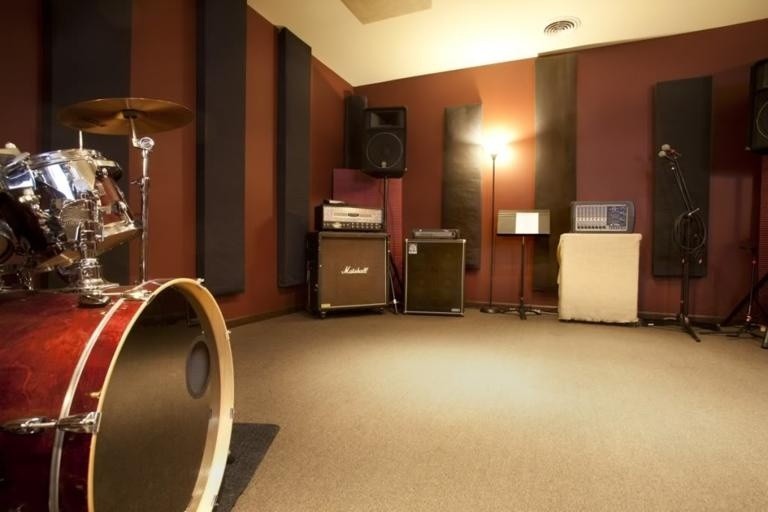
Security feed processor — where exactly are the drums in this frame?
[1,278,234,510]
[3,149,141,274]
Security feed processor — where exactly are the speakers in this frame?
[403,239,467,316]
[305,231,386,318]
[747,58,768,156]
[360,106,407,179]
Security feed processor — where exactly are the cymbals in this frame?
[61,98,194,137]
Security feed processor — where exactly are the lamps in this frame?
[480,142,511,316]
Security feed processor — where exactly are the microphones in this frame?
[657,150,674,162]
[661,143,681,157]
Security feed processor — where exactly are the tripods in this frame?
[384,177,402,314]
[500,236,541,319]
[643,163,720,341]
[700,247,765,339]
